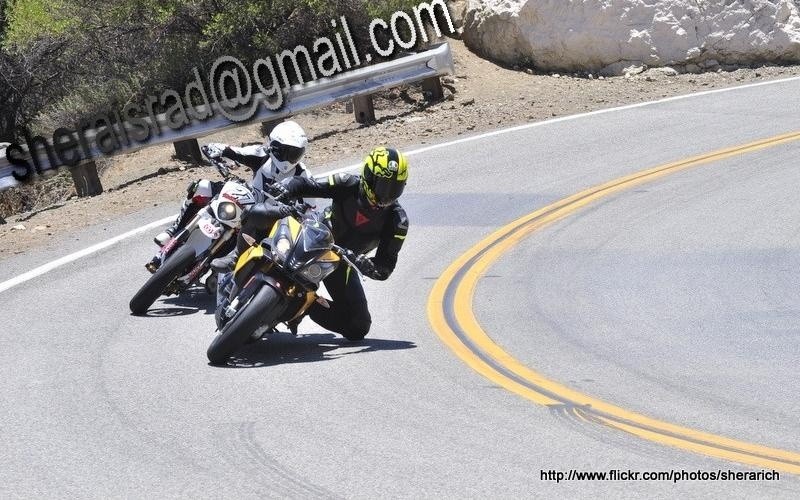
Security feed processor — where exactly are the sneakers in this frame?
[154,224,178,248]
[211,250,240,268]
[288,309,305,335]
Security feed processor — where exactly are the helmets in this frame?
[361,146,409,208]
[268,120,307,174]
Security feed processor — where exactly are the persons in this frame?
[209,146,410,340]
[153,121,316,295]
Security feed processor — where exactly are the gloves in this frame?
[354,253,376,275]
[270,182,292,203]
[205,142,229,158]
